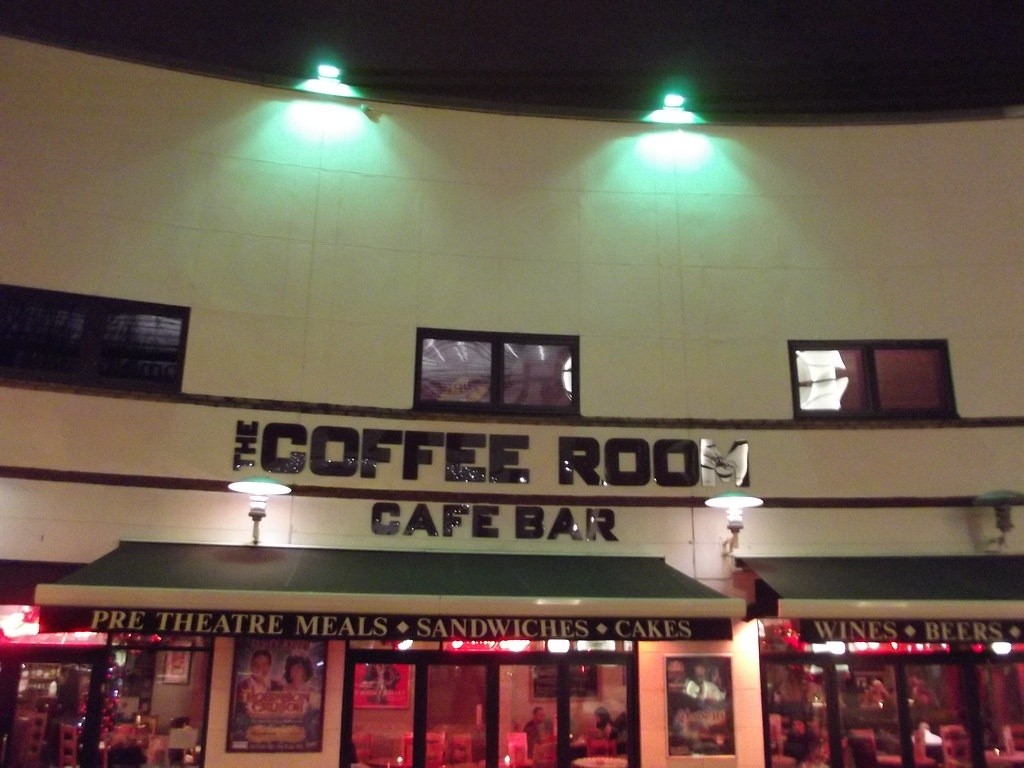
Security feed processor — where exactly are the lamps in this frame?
[227,477,292,543]
[976,489,1024,544]
[705,492,764,557]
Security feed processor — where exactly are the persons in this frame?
[39,665,204,768]
[668,658,732,756]
[767,673,993,768]
[521,706,628,759]
[233,650,321,742]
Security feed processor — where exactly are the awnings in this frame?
[33,541,746,642]
[736,556,1024,621]
